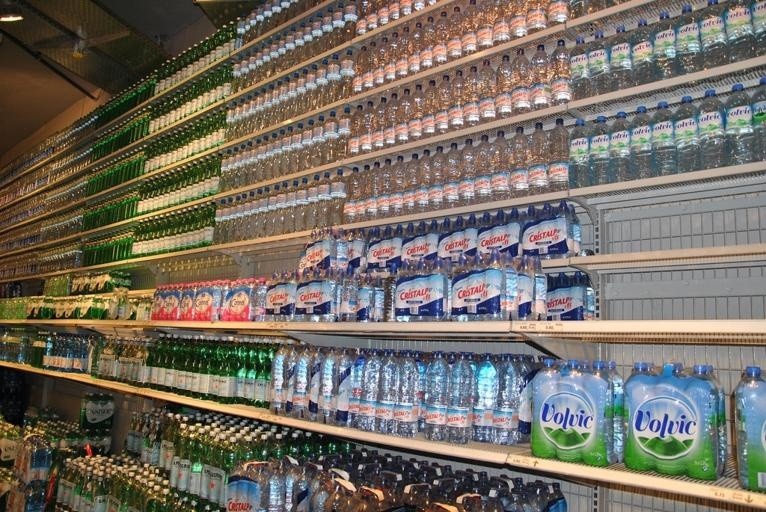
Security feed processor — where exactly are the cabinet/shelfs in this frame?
[0,1,766,512]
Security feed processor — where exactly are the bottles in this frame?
[0,0,765,512]
